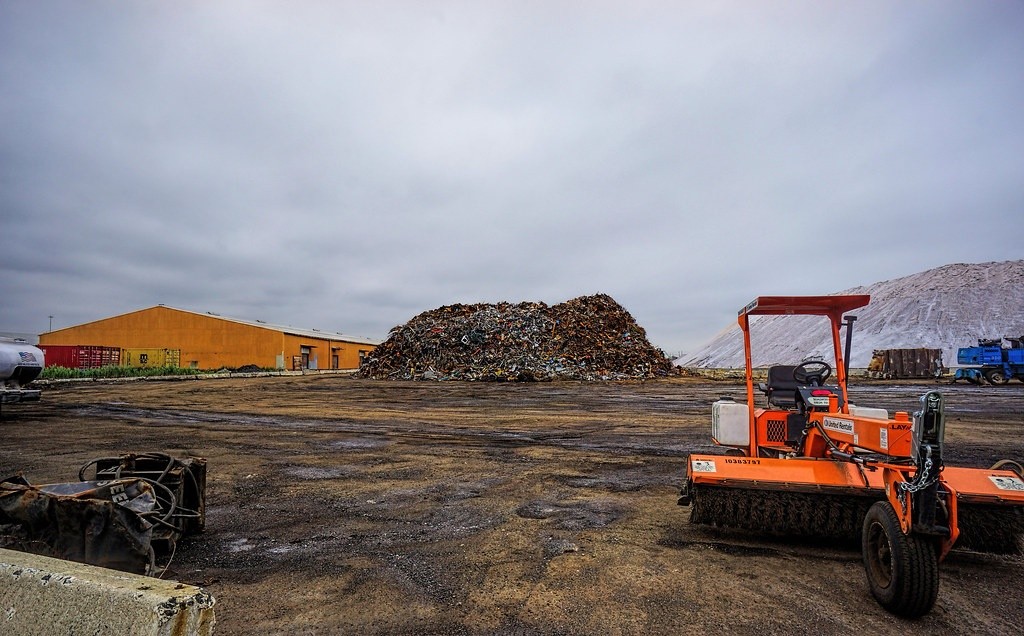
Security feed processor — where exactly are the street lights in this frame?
[49,315,54,333]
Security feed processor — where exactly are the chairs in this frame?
[764,364,810,408]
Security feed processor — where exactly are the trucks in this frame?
[956,335,1024,386]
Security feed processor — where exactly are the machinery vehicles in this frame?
[680,294,1024,622]
